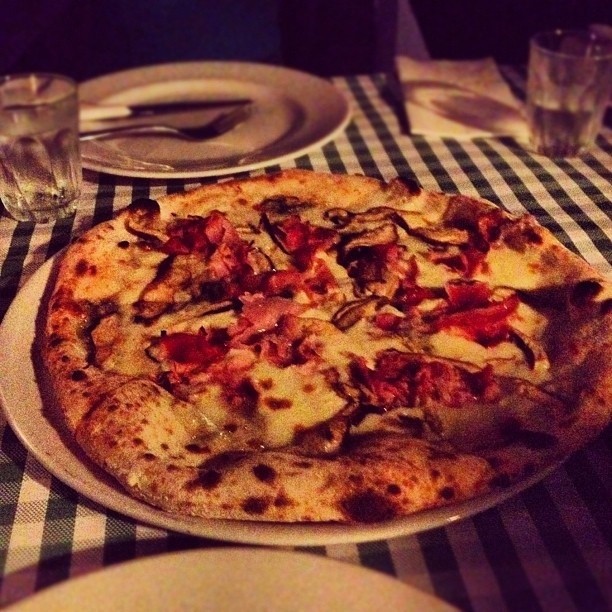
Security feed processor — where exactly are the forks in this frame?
[78,106,254,142]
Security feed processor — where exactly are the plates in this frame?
[78,61,355,179]
[1,546,466,611]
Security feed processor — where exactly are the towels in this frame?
[391,53,529,139]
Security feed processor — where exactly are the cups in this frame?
[0,71,80,221]
[526,23,612,156]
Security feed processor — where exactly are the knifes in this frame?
[78,95,255,121]
